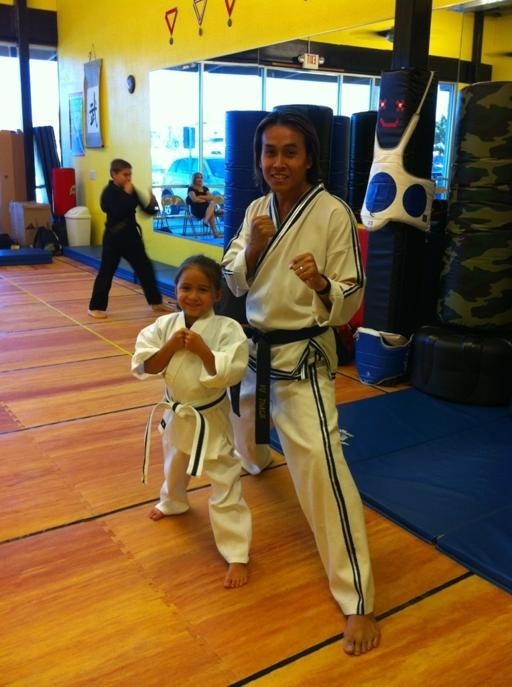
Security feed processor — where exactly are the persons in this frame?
[188,171,221,237]
[129,253,253,588]
[86,157,176,319]
[220,109,384,657]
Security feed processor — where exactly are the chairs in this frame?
[153,195,225,237]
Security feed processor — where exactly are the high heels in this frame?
[203,222,208,227]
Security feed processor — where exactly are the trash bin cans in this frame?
[64,206,91,246]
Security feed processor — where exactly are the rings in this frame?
[299,265,303,272]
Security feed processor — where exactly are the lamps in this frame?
[297,54,325,69]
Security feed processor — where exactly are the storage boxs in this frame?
[8,201,52,249]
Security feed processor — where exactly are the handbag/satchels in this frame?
[186,192,198,205]
[33,223,67,256]
[0,234,18,249]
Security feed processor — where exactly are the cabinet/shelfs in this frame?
[0,133,27,235]
